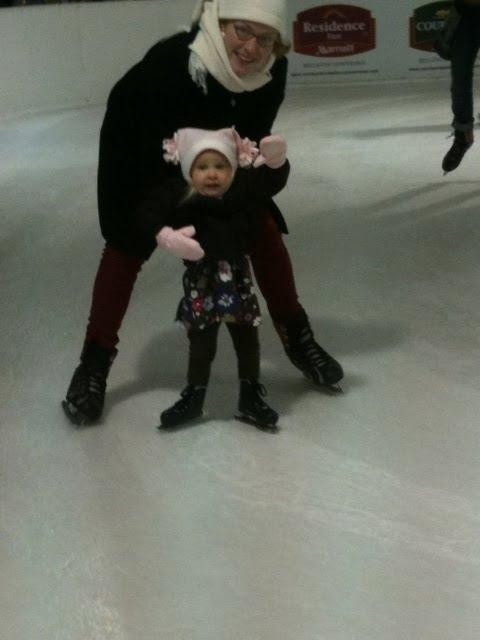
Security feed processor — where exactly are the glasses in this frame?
[221,23,281,50]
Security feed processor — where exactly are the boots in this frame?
[64,340,120,422]
[442,120,475,171]
[270,307,344,387]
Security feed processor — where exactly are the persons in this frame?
[62,0,343,426]
[438,0,480,175]
[156,121,292,434]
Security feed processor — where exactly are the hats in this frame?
[171,126,239,186]
[214,0,292,55]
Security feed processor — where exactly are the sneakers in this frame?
[237,381,279,425]
[159,387,207,429]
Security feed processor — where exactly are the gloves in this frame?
[156,223,206,263]
[259,132,288,170]
